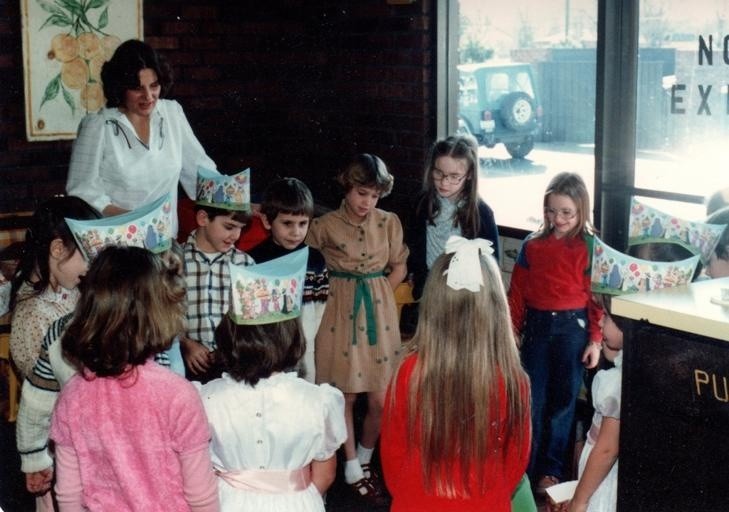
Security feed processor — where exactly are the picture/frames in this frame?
[20,0,145,142]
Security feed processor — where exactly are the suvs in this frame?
[454,60,545,158]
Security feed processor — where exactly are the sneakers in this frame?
[535,477,558,493]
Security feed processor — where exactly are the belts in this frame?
[529,310,588,318]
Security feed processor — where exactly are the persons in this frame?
[565,188,728,512]
[9,192,105,384]
[190,246,349,512]
[380,235,533,511]
[177,164,257,381]
[16,189,179,511]
[404,136,501,338]
[509,170,604,497]
[303,152,411,505]
[48,244,219,512]
[65,39,222,218]
[244,177,330,386]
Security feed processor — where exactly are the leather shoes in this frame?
[345,463,385,501]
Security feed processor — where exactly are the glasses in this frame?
[432,169,468,185]
[544,207,579,219]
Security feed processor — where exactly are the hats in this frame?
[196,165,251,212]
[628,199,728,265]
[591,234,701,296]
[65,192,171,265]
[228,247,309,325]
